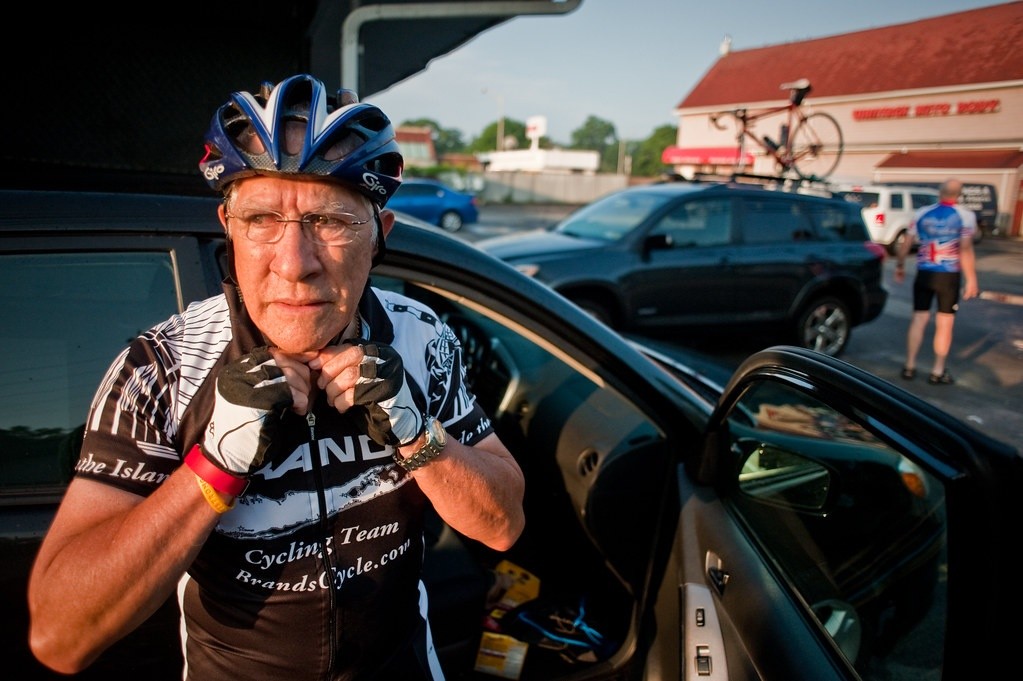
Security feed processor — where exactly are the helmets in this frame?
[197,71,404,211]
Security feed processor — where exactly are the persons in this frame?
[26,73,526,681]
[893,179,978,385]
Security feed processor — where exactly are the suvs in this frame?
[831,183,980,254]
[0,186,1023,680]
[467,179,891,357]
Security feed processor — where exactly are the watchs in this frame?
[896,261,903,269]
[392,415,447,471]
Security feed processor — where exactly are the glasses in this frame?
[223,210,377,246]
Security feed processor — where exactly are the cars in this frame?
[389,179,479,232]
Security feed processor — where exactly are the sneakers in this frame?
[928,367,956,385]
[900,365,918,380]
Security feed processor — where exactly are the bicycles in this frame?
[701,78,843,181]
